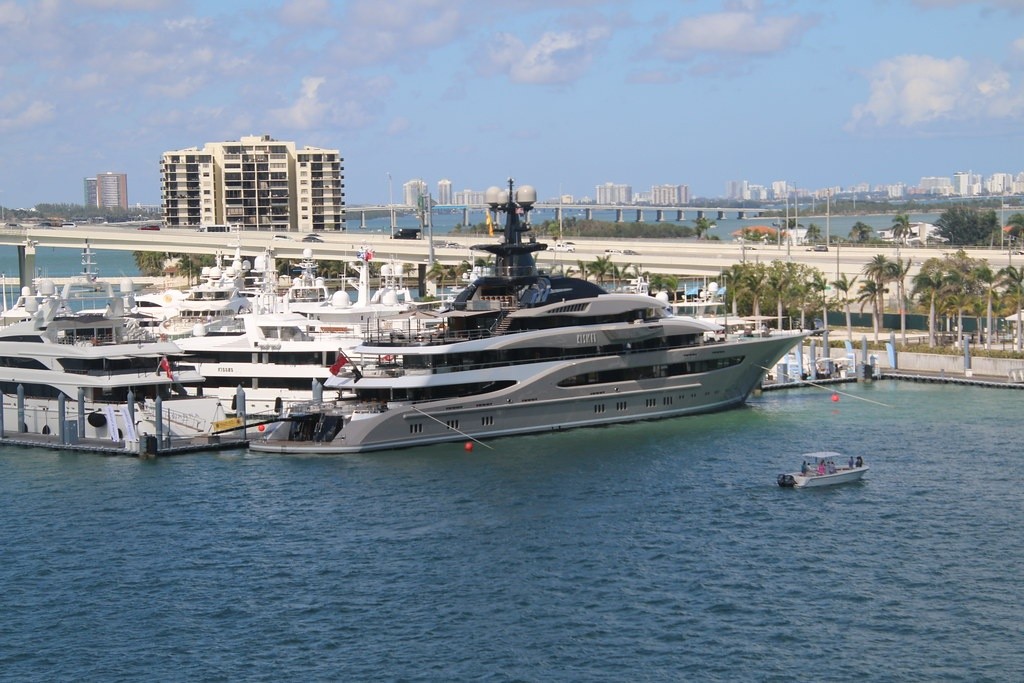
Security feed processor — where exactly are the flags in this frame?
[330,354,348,375]
[160,357,174,381]
[357,251,366,259]
[365,252,373,262]
[486,208,495,236]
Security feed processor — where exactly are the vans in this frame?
[197,225,231,232]
[390,228,421,240]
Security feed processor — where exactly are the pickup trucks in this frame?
[431,238,466,248]
[548,243,575,252]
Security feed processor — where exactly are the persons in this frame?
[801,456,864,475]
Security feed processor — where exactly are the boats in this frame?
[1,177,835,459]
[777,451,870,488]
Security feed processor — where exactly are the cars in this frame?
[306,233,320,237]
[137,225,160,230]
[302,237,323,243]
[273,234,294,241]
[740,246,757,251]
[805,244,828,252]
[605,249,622,254]
[624,250,640,254]
